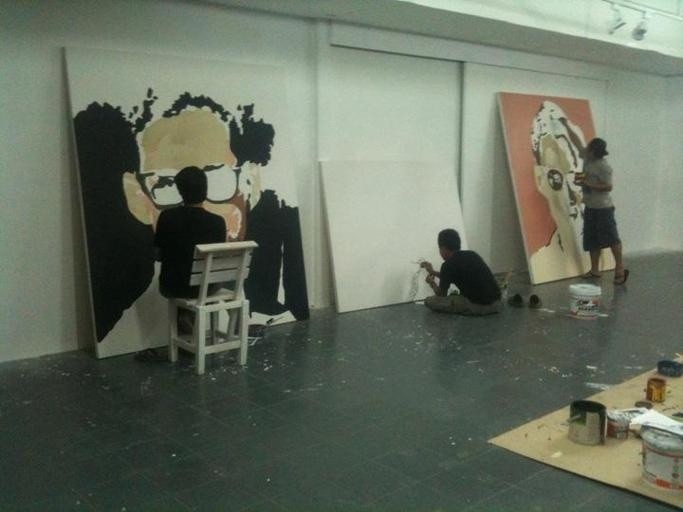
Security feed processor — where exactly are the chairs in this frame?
[168,241,259,376]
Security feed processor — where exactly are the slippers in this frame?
[529,295,542,308]
[508,294,524,307]
[614,269,629,284]
[584,270,602,278]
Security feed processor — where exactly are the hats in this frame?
[588,138,609,155]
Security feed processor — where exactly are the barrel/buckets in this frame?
[568,400,606,445]
[647,378,666,404]
[640,417,683,492]
[607,412,630,439]
[569,284,602,317]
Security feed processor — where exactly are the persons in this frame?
[558,116,630,286]
[420,228,505,314]
[97,93,298,354]
[154,166,227,353]
[529,100,616,282]
